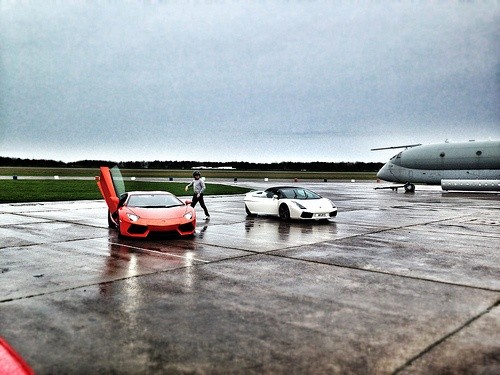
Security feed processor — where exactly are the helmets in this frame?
[193,171,202,177]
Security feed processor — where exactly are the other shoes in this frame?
[205,215,210,220]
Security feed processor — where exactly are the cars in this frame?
[94,165,197,241]
[244,186,338,223]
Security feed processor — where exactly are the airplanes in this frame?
[369,141,500,197]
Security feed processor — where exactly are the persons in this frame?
[184,171,211,220]
